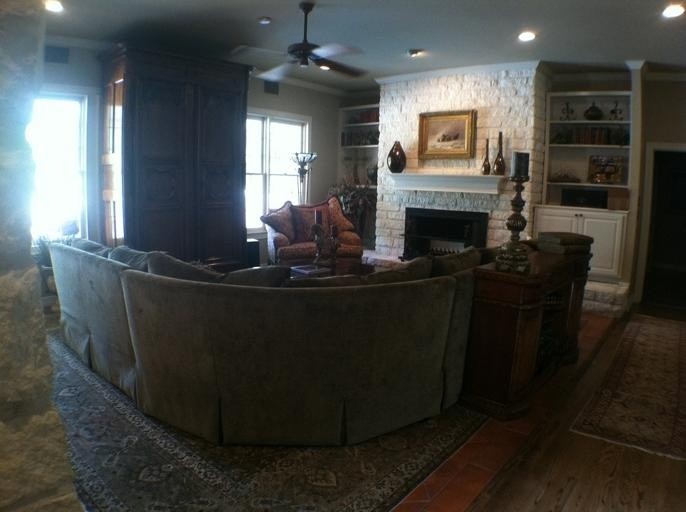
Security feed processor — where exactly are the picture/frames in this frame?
[417,109,477,160]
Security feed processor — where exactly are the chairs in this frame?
[259,196,349,265]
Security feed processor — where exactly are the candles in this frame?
[510,151,531,178]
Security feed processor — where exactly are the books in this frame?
[548,125,628,144]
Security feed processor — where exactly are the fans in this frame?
[229,0,368,86]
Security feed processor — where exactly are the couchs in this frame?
[44,235,540,446]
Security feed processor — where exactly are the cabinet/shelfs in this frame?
[461,251,593,419]
[99,42,255,273]
[541,88,630,213]
[333,105,380,251]
[531,203,628,281]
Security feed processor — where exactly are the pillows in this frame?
[260,195,357,241]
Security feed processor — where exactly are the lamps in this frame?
[289,152,317,203]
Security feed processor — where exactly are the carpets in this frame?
[43,329,493,512]
[570,310,686,463]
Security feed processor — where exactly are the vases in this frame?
[480,139,490,174]
[492,130,507,175]
[388,141,408,174]
